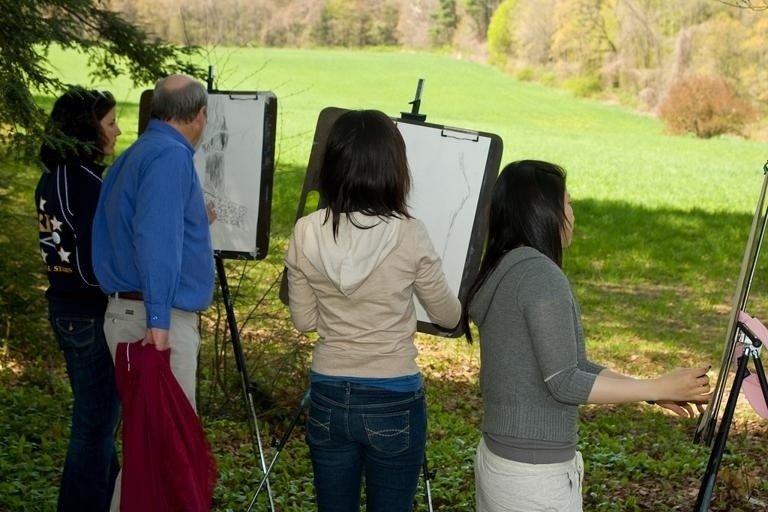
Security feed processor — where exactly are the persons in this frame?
[286,110,463,512]
[34,90,215,512]
[92,76,216,512]
[463,160,711,512]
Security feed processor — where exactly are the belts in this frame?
[111,291,144,300]
